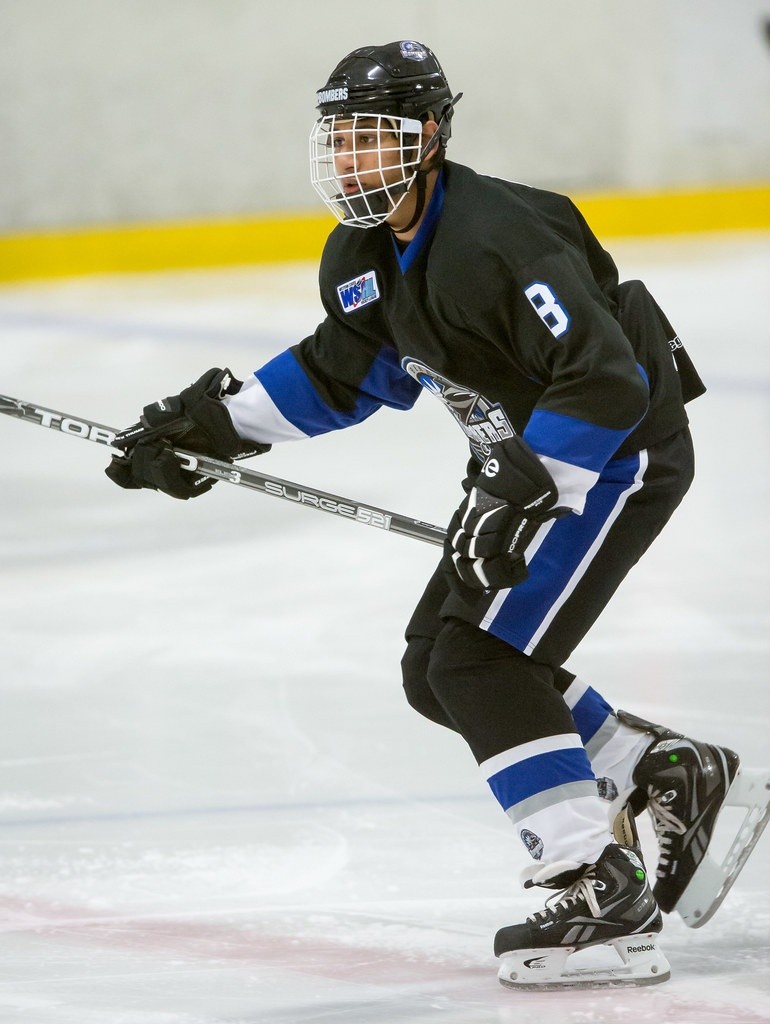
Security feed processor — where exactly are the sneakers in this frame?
[492,802,672,992]
[612,708,770,928]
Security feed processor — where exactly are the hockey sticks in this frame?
[0,394,448,549]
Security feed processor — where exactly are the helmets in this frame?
[316,41,455,143]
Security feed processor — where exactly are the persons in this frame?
[104,41,770,990]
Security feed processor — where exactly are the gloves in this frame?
[104,365,273,501]
[441,433,559,605]
